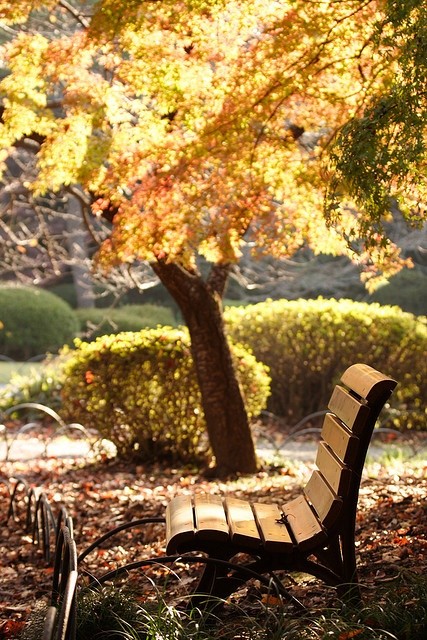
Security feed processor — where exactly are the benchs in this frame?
[166,363,397,610]
